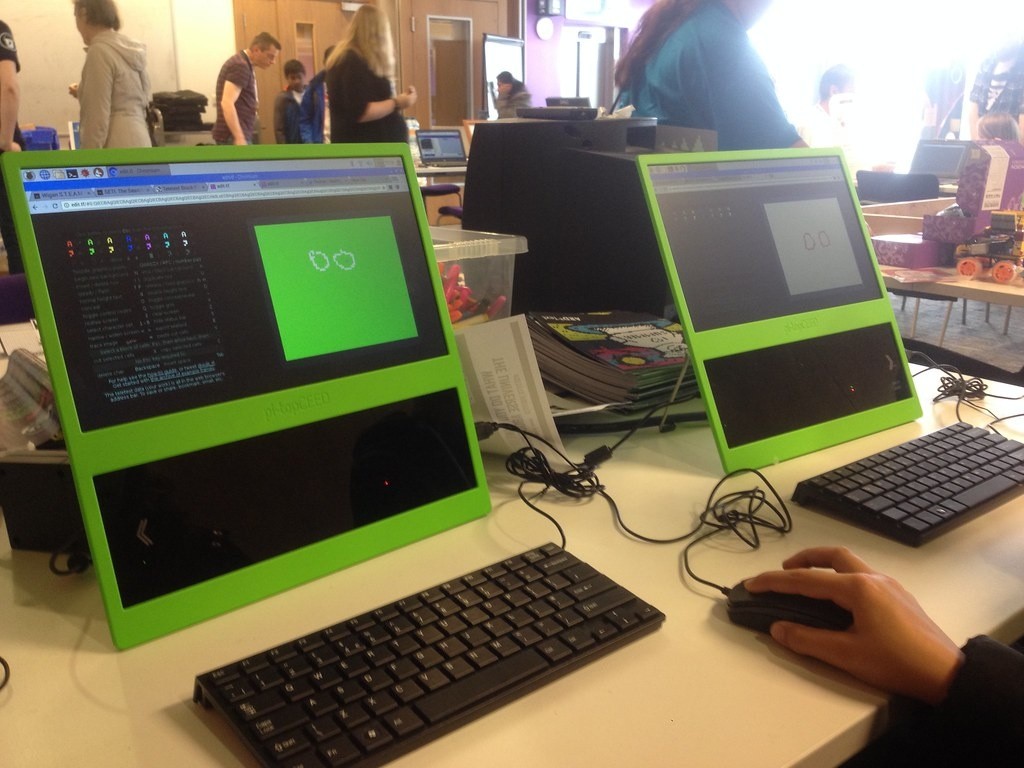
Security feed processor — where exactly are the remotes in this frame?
[516,106,598,120]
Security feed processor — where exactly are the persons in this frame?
[69,0,152,149]
[273,59,314,144]
[743,545,1024,768]
[615,0,810,152]
[495,71,531,119]
[921,42,1024,216]
[212,32,281,145]
[299,45,335,144]
[802,64,867,181]
[325,4,417,143]
[0,20,26,275]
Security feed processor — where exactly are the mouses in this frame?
[726,578,855,632]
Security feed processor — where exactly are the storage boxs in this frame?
[860,197,956,237]
[921,140,1024,246]
[870,235,955,269]
[425,226,529,332]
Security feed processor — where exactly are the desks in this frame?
[0,257,1024,767]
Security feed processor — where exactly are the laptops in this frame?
[416,129,469,167]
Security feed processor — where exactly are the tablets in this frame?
[0,143,492,652]
[634,146,923,475]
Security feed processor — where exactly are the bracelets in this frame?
[391,97,401,113]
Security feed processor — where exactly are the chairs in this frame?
[857,171,959,345]
[419,182,463,227]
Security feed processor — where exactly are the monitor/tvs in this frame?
[905,137,972,186]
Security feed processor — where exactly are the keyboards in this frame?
[791,421,1024,548]
[192,542,666,768]
